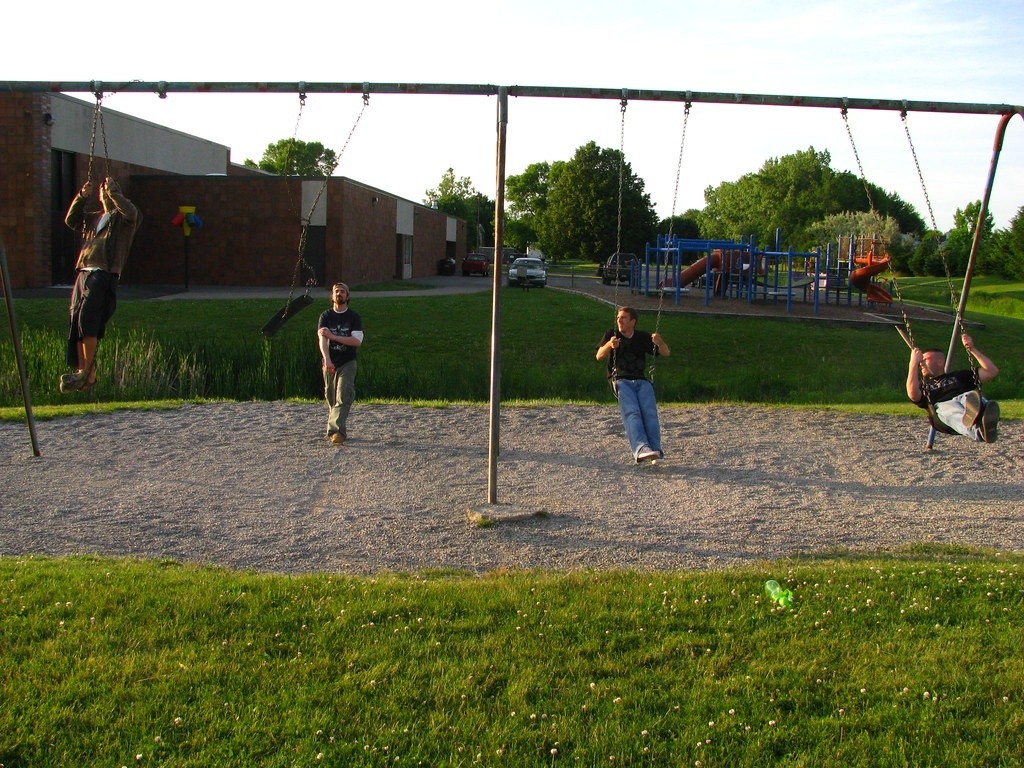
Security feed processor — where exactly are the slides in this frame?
[658,253,721,288]
[851,258,894,304]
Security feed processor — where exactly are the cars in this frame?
[462,253,491,277]
[508,257,547,288]
[692,271,717,289]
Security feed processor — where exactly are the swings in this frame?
[77,93,111,391]
[261,92,369,337]
[612,99,691,398]
[841,107,983,434]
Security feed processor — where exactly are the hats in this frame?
[331,282,350,305]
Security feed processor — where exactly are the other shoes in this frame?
[980,401,1000,443]
[962,390,982,428]
[59,369,98,394]
[637,445,660,460]
[652,458,664,465]
[330,432,345,444]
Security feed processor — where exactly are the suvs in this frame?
[603,252,642,285]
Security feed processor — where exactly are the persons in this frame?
[58,176,137,394]
[903,334,1001,445]
[595,306,670,465]
[317,283,364,443]
